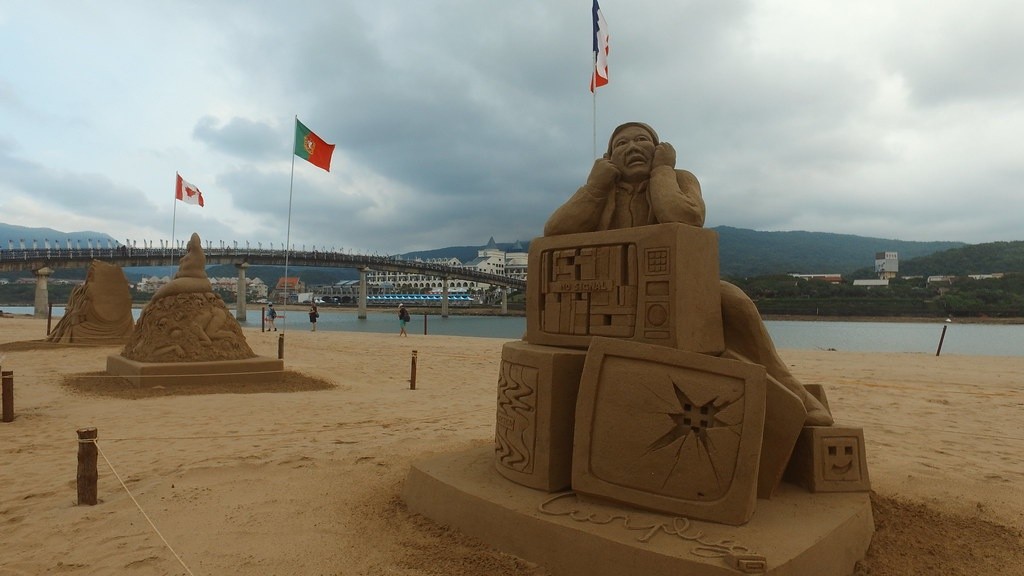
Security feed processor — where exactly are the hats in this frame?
[269,302,272,305]
[398,304,403,308]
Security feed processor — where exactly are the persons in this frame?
[266,302,277,332]
[174,231,207,278]
[398,303,410,337]
[308,301,318,331]
[121,245,126,255]
[543,121,833,425]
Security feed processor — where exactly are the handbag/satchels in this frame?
[315,312,319,318]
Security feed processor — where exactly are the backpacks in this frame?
[404,312,410,322]
[270,308,276,318]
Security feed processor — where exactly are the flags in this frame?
[590,0,610,95]
[294,119,336,172]
[175,174,204,207]
